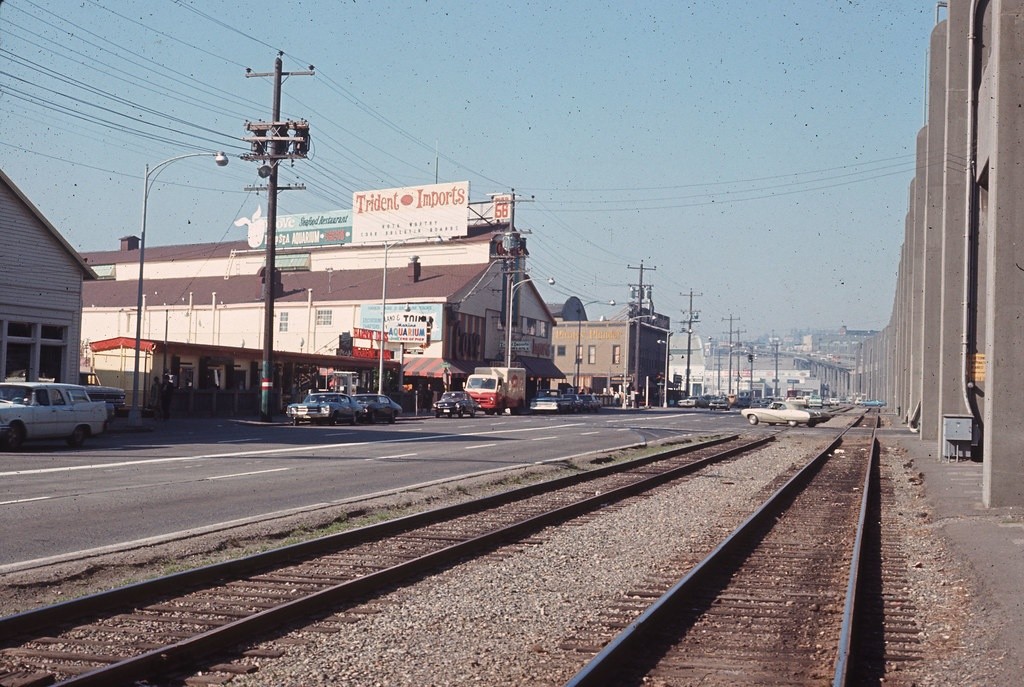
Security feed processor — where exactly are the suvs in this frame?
[79,372,125,410]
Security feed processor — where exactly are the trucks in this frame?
[463,367,525,415]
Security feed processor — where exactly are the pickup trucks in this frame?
[529,390,571,414]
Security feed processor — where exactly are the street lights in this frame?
[576,300,615,394]
[702,335,712,394]
[663,327,693,407]
[127,152,229,429]
[508,277,556,367]
[727,349,746,392]
[624,316,656,400]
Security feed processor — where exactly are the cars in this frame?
[431,392,478,418]
[861,399,887,407]
[855,397,862,405]
[562,394,583,412]
[286,393,364,426]
[740,401,831,429]
[348,395,401,425]
[708,398,730,411]
[0,382,115,448]
[736,396,840,410]
[579,394,598,412]
[678,396,707,408]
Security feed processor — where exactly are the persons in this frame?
[151,376,161,421]
[569,387,593,394]
[25,389,33,400]
[614,391,624,407]
[424,388,434,413]
[161,375,174,422]
